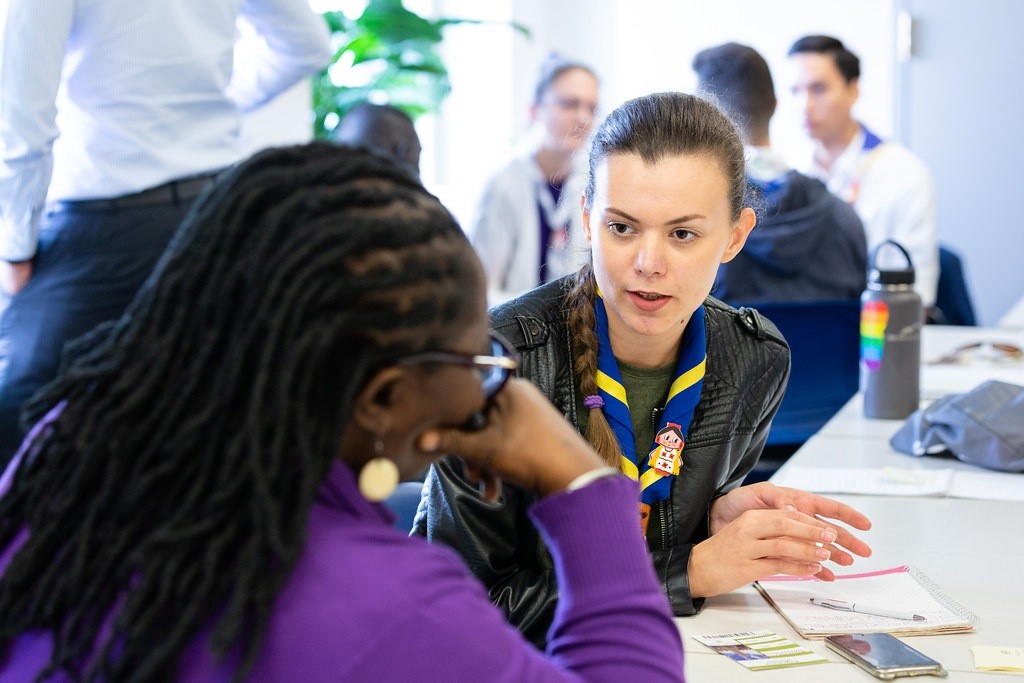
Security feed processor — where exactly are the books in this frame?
[752,563,980,639]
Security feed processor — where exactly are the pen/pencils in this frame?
[811,596,929,622]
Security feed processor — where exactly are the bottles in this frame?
[861,237,924,420]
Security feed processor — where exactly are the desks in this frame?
[673,325,1024,683]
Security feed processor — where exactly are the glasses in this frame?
[399,328,524,370]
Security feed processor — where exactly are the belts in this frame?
[67,175,215,210]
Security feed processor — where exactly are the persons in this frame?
[0,0,938,683]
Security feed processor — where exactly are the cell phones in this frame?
[824,632,942,679]
